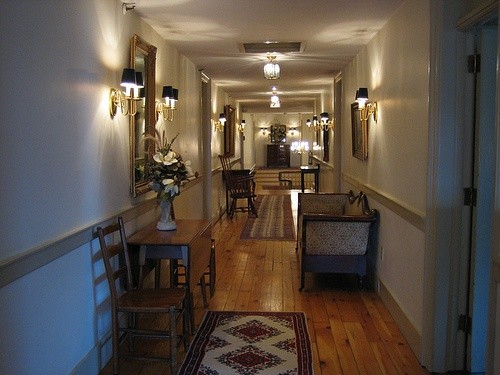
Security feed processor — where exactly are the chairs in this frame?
[96,216,189,375]
[164,201,216,296]
[296,190,377,293]
[219,154,258,219]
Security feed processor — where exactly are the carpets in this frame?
[240,194,297,241]
[175,310,313,375]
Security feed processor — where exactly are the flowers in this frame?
[140,128,200,197]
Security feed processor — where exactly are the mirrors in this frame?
[270,124,286,143]
[130,35,156,198]
[224,104,235,158]
[349,102,368,161]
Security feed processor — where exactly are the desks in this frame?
[223,162,256,213]
[301,165,319,193]
[123,217,212,333]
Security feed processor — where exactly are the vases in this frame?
[156,193,176,231]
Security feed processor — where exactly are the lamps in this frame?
[260,127,268,137]
[108,67,144,116]
[271,95,282,109]
[305,112,334,131]
[354,87,377,121]
[239,120,246,133]
[155,85,178,122]
[214,113,226,131]
[263,55,280,79]
[289,127,296,136]
[139,87,146,108]
